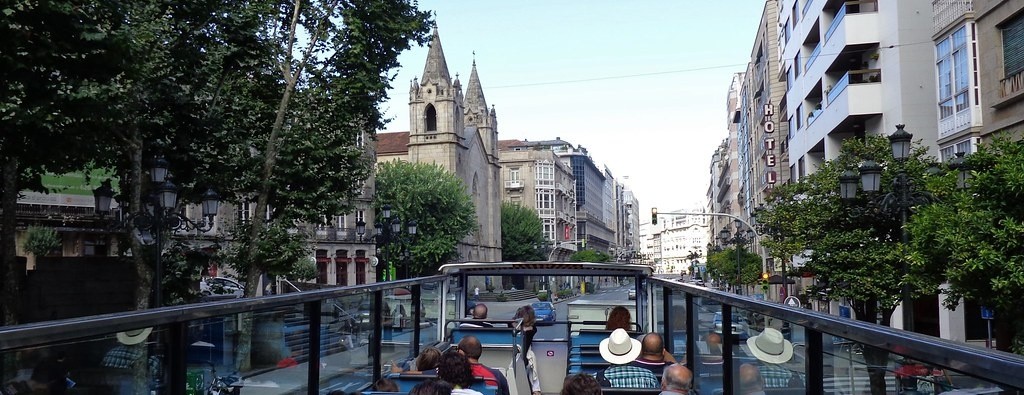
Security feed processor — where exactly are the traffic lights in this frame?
[562,223,570,240]
[652,208,657,225]
[762,272,770,293]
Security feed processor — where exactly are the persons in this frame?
[590,328,662,389]
[631,333,674,385]
[560,374,602,395]
[739,363,765,395]
[473,284,481,302]
[48,351,67,395]
[459,304,493,328]
[98,326,158,378]
[747,327,806,388]
[383,317,393,340]
[720,283,731,292]
[672,305,686,330]
[577,281,580,289]
[752,316,773,326]
[558,280,570,290]
[372,377,399,392]
[390,334,511,395]
[680,331,724,365]
[605,306,632,331]
[383,301,407,317]
[507,306,537,395]
[658,363,692,395]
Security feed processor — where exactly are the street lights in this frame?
[355,203,417,387]
[720,215,756,295]
[836,122,974,395]
[91,158,220,395]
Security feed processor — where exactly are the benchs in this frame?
[388,371,503,395]
[444,317,524,354]
[360,390,409,395]
[564,317,672,395]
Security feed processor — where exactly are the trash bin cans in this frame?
[186,367,204,395]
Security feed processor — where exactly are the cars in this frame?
[199,276,244,300]
[628,286,636,300]
[714,312,746,339]
[353,301,391,329]
[465,300,477,315]
[532,302,557,326]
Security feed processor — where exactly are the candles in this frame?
[557,289,573,298]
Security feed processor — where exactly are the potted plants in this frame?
[815,103,822,110]
[869,53,879,60]
[537,292,547,301]
[862,61,869,67]
[496,290,508,302]
[808,112,814,117]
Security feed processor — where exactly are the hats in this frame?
[747,328,793,363]
[599,328,642,364]
[116,326,153,345]
